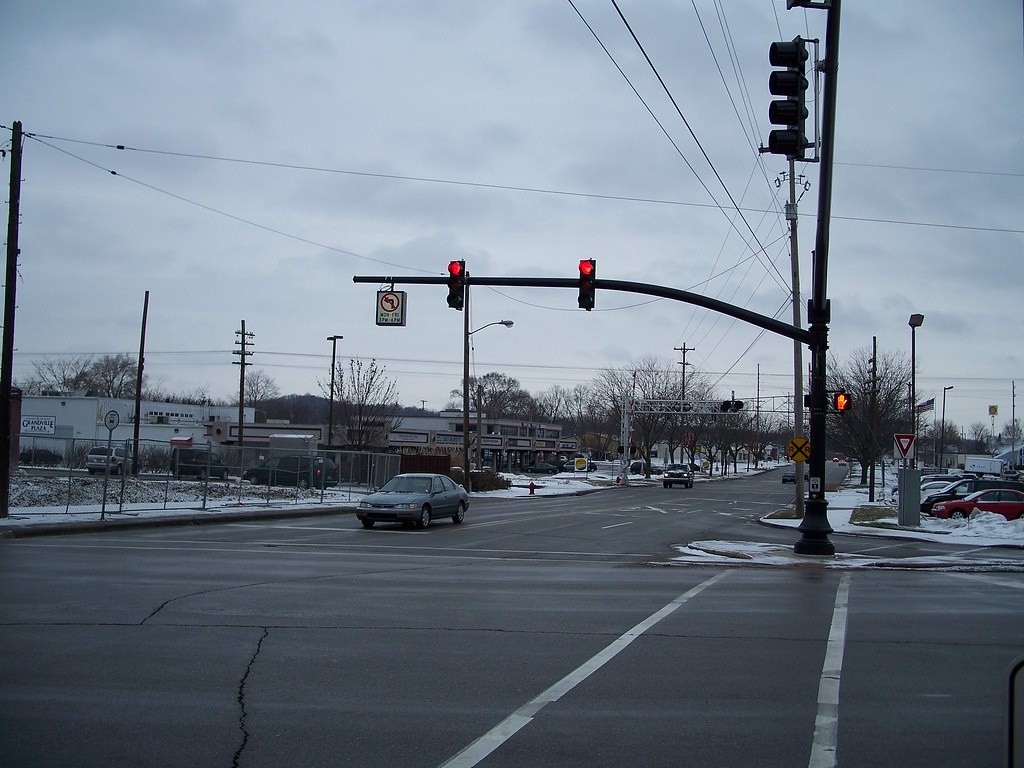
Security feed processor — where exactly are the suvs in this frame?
[242,455,339,490]
[920,479,1024,516]
[86,445,142,474]
[1002,470,1024,479]
[663,463,695,488]
[170,447,229,481]
[629,462,663,475]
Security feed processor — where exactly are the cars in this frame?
[782,472,797,484]
[524,463,558,474]
[930,489,1024,520]
[560,456,597,473]
[805,471,810,481]
[833,458,840,461]
[920,469,1001,501]
[354,473,470,529]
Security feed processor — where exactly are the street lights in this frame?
[939,385,954,474]
[463,319,514,494]
[326,335,345,449]
[897,312,925,530]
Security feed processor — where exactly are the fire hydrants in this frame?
[529,482,536,495]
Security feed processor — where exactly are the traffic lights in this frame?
[835,393,852,412]
[578,259,597,311]
[769,36,811,162]
[446,259,466,309]
